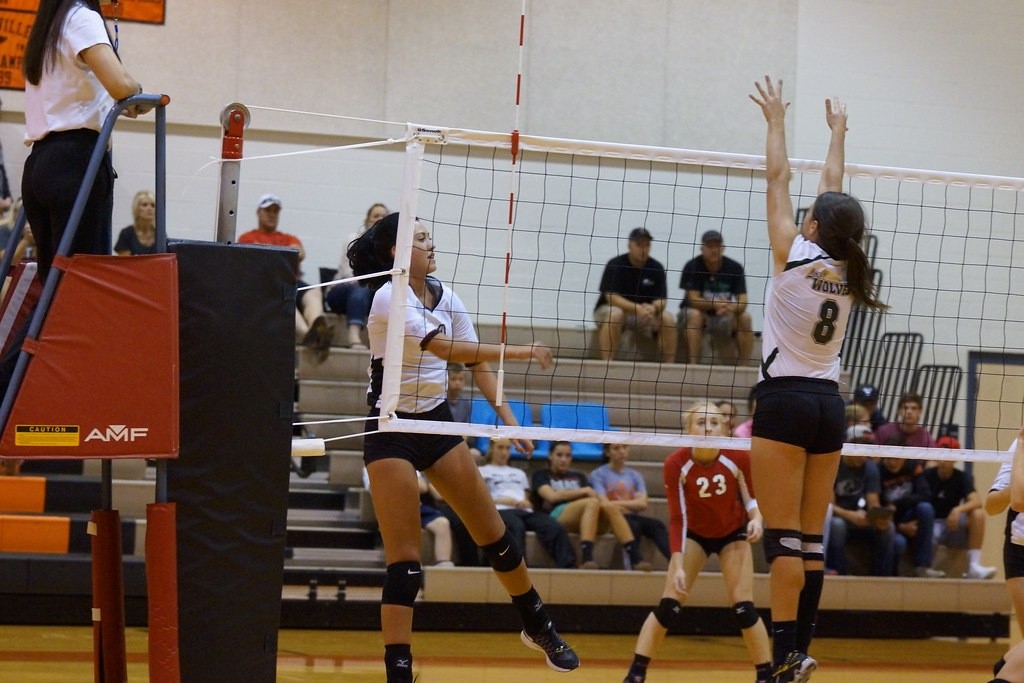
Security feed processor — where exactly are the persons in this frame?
[749,75,892,683]
[624,402,772,683]
[237,198,389,351]
[0,0,143,408]
[114,190,156,256]
[362,362,671,569]
[347,212,580,683]
[593,228,754,366]
[0,160,38,277]
[985,428,1024,683]
[716,384,996,579]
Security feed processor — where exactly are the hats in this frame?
[847,424,873,440]
[933,435,960,448]
[629,227,654,241]
[854,383,880,403]
[703,230,723,243]
[256,195,281,213]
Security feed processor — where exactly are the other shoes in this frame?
[634,561,652,570]
[302,314,335,361]
[347,342,367,350]
[579,561,598,569]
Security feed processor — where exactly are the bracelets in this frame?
[138,84,142,94]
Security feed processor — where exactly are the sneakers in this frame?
[521,622,579,671]
[912,565,946,578]
[773,653,817,683]
[967,563,997,579]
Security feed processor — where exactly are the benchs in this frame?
[297,305,1014,618]
